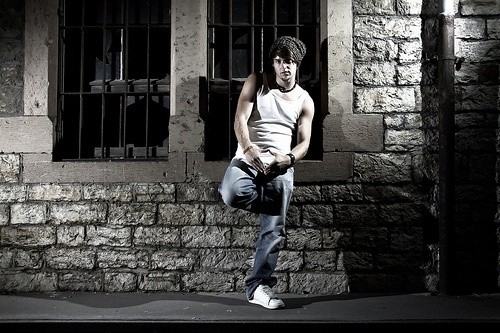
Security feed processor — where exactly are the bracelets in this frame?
[285,153,295,166]
[243,145,253,154]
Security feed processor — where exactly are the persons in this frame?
[221,36,314,310]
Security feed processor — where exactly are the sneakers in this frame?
[246,284,285,309]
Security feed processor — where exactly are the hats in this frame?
[269,36,306,66]
